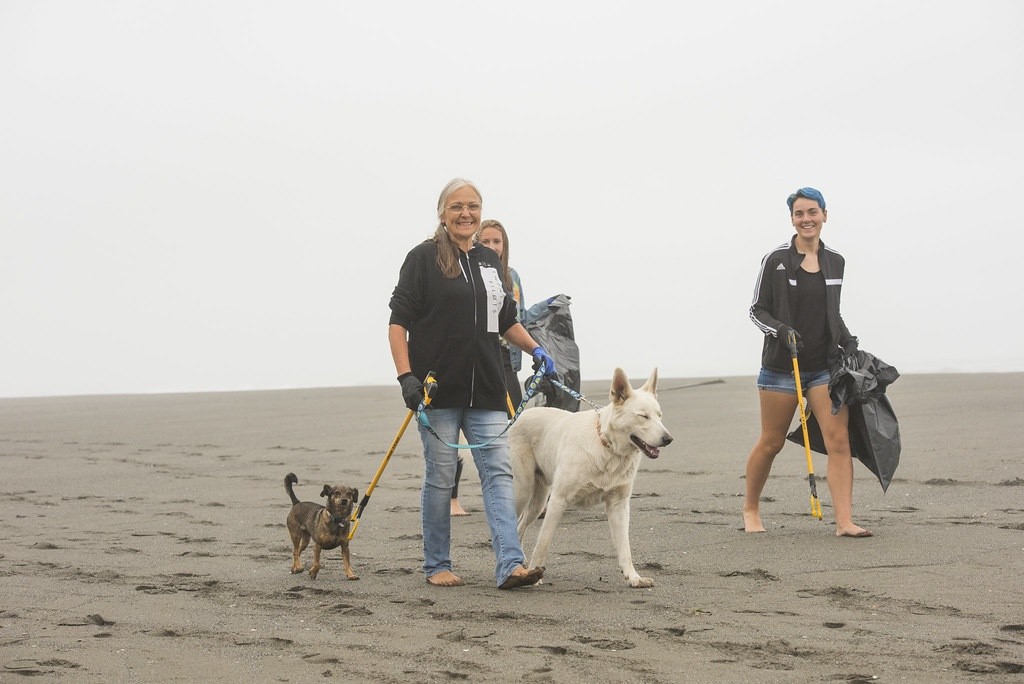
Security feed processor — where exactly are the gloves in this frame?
[398,371,434,411]
[532,346,554,376]
[776,323,804,353]
[845,340,857,353]
[546,294,572,312]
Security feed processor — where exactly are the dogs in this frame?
[508,366,673,587]
[283,472,360,581]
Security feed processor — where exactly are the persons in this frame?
[743,187,873,537]
[448,220,525,517]
[387,179,553,589]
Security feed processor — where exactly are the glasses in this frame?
[444,203,482,214]
